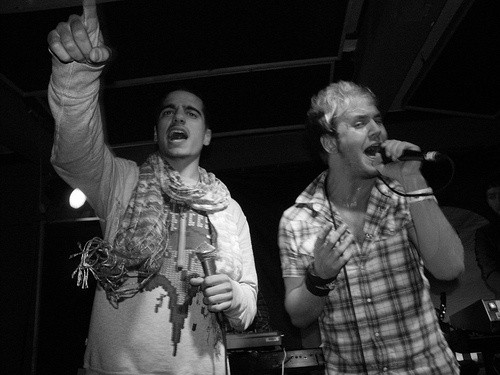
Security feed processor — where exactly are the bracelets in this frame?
[406,187,436,203]
[304,264,337,297]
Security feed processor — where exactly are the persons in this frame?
[43,0,258,375]
[277,80,466,375]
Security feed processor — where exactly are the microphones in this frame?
[195,240,225,331]
[378,147,441,165]
